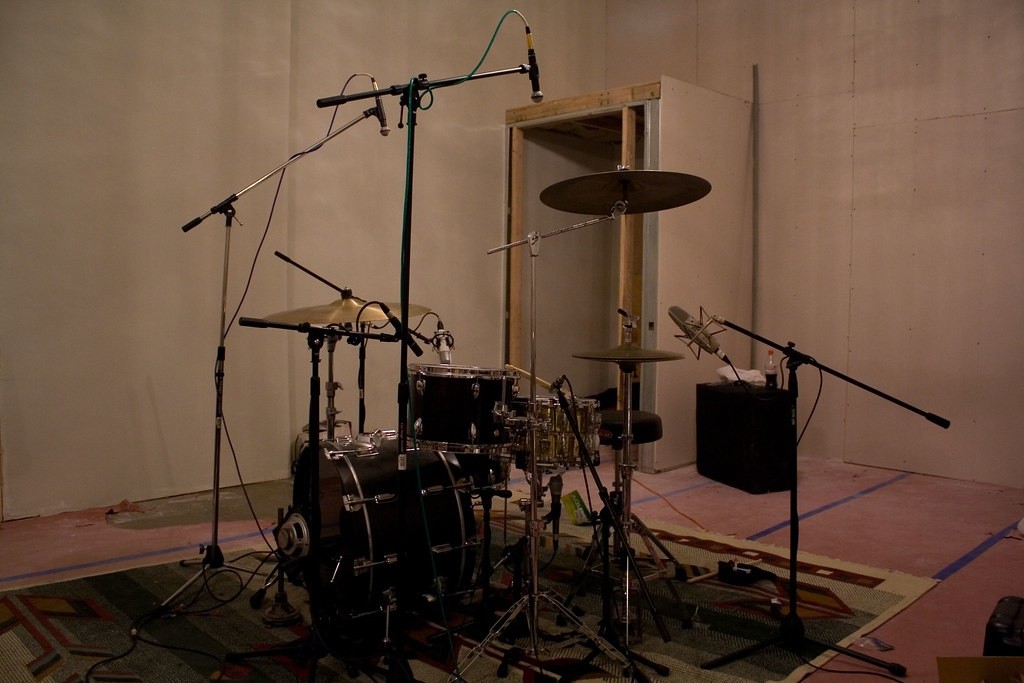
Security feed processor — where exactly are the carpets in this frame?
[0,513,941,683]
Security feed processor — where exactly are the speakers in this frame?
[695,382,794,495]
[982,596,1024,658]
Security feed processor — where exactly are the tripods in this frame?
[134,109,952,683]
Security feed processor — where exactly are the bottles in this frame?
[765,351,779,390]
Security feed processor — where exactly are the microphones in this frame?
[436,320,450,364]
[669,306,731,365]
[380,302,423,358]
[525,26,543,104]
[370,77,390,136]
[548,375,566,393]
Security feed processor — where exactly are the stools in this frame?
[696,381,795,492]
[555,408,693,633]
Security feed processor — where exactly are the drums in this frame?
[292,429,479,637]
[513,395,601,471]
[467,453,512,494]
[406,363,521,455]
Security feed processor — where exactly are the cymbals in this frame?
[571,341,687,368]
[262,298,432,325]
[538,167,712,216]
